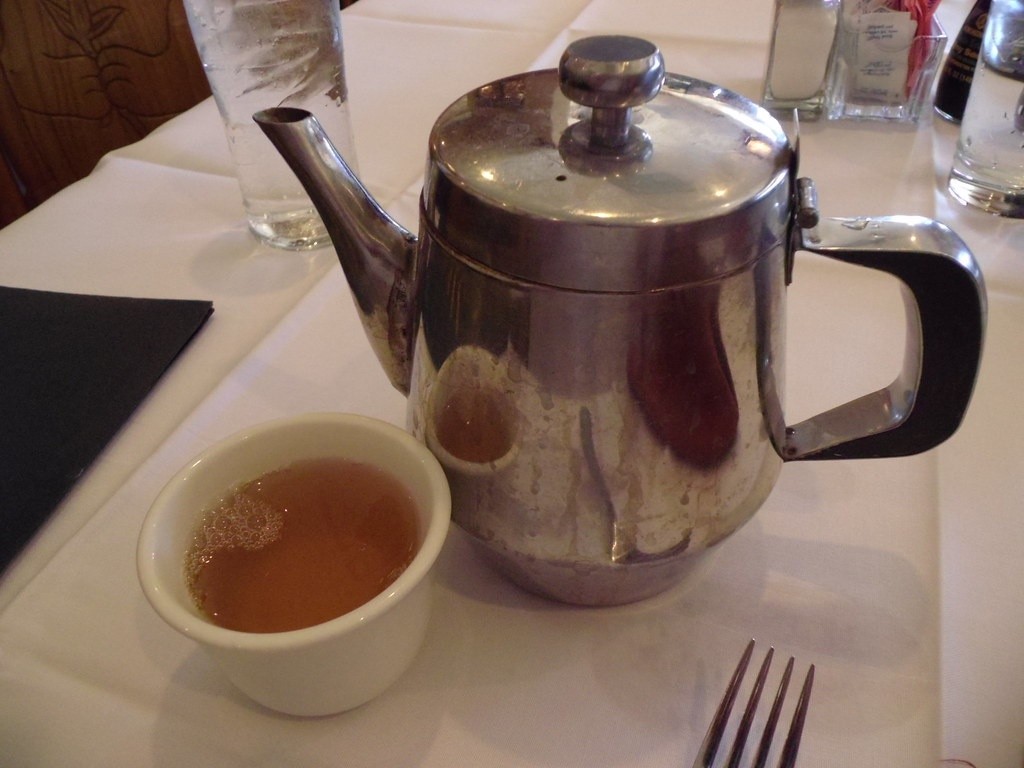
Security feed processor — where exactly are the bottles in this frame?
[933,1,995,124]
[946,0,1024,222]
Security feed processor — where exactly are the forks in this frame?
[693,639,816,768]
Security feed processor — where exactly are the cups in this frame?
[180,0,359,252]
[136,414,451,718]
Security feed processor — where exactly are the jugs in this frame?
[252,34,989,606]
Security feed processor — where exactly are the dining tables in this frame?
[0,0,1024,768]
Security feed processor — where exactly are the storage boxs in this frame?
[823,10,949,124]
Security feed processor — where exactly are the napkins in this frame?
[0,278,220,577]
